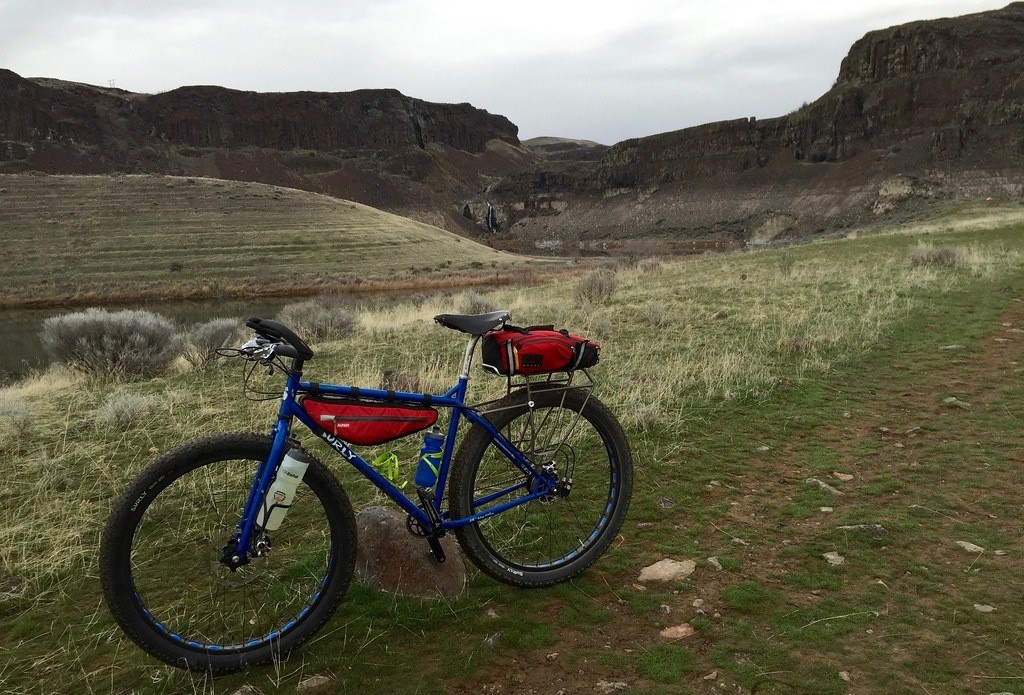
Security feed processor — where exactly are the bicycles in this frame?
[97,309,634,677]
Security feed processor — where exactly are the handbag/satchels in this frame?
[481,324,601,375]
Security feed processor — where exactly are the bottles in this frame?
[414,426,446,487]
[255,433,311,532]
